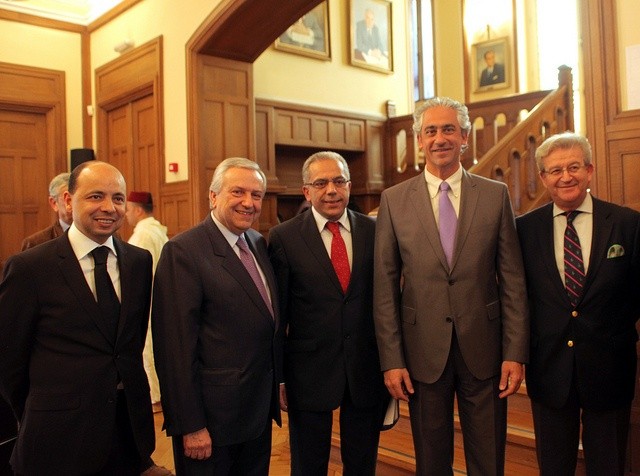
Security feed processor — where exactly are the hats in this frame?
[128,191,153,204]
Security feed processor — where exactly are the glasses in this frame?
[302,178,351,189]
[543,165,587,176]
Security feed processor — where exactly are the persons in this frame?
[268,151,391,476]
[355,7,384,59]
[511,130,640,476]
[151,154,278,475]
[2,157,157,474]
[123,188,169,414]
[373,97,528,475]
[279,17,316,51]
[479,49,506,86]
[22,172,75,249]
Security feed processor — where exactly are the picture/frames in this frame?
[470,37,512,94]
[275,1,333,63]
[347,0,396,76]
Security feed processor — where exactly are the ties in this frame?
[326,221,350,294]
[89,244,123,361]
[560,210,586,309]
[235,236,275,320]
[436,181,458,268]
